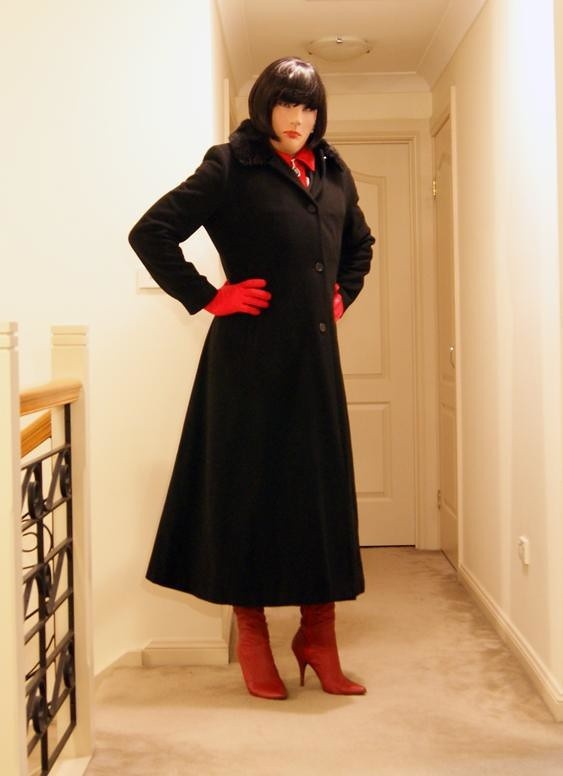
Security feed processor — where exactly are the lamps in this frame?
[306,35,373,63]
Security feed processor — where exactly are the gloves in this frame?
[334,283,344,320]
[203,279,272,316]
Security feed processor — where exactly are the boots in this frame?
[292,602,367,695]
[233,605,289,699]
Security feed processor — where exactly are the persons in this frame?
[127,56,376,700]
